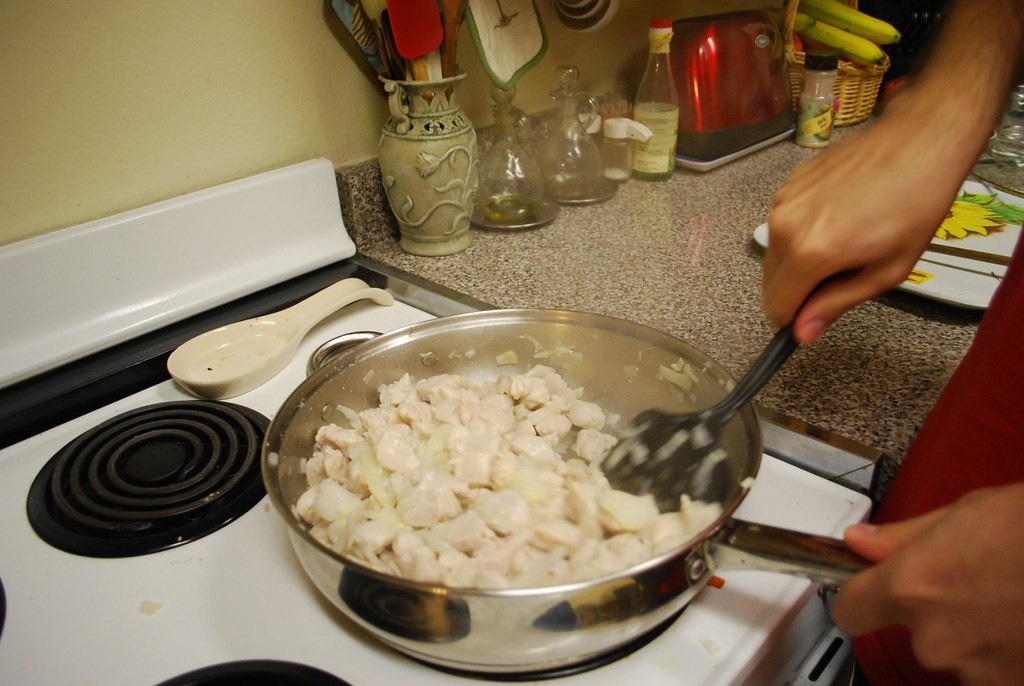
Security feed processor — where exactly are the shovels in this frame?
[600,325,798,514]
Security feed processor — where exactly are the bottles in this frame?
[794,49,839,150]
[632,18,679,181]
[477,83,544,222]
[536,62,602,199]
[377,69,478,257]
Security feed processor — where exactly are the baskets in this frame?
[783,0,889,126]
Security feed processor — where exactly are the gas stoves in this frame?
[0,158,878,685]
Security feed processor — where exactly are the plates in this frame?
[471,202,560,229]
[754,179,1024,309]
[553,177,619,203]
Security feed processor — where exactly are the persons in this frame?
[761,0,1024,686]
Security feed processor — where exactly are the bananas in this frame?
[762,1,903,68]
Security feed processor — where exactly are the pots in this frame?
[261,306,881,675]
